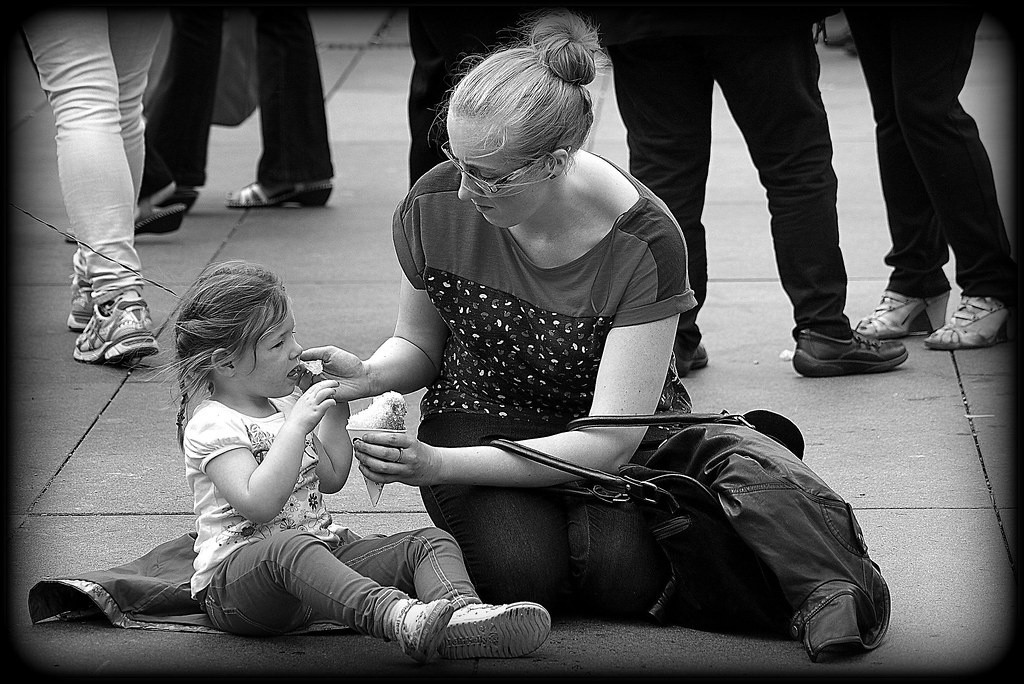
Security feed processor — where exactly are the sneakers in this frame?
[674,342,708,377]
[73,296,160,366]
[793,320,908,378]
[67,298,94,332]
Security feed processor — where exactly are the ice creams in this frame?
[348,390,406,431]
[306,359,323,375]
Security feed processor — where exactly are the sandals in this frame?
[225,179,333,208]
[66,179,186,241]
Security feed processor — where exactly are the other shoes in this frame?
[395,598,454,664]
[183,190,198,213]
[823,10,851,46]
[436,602,552,660]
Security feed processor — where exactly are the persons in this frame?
[404,0,538,190]
[134,0,335,235]
[595,0,909,375]
[299,10,891,656]
[841,0,1014,349]
[175,258,553,660]
[9,0,173,363]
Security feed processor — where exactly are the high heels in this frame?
[855,290,950,339]
[924,296,1011,350]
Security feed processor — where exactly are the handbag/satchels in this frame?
[142,9,259,127]
[490,409,804,638]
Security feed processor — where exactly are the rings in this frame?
[396,447,403,463]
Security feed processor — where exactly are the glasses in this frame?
[441,141,572,194]
